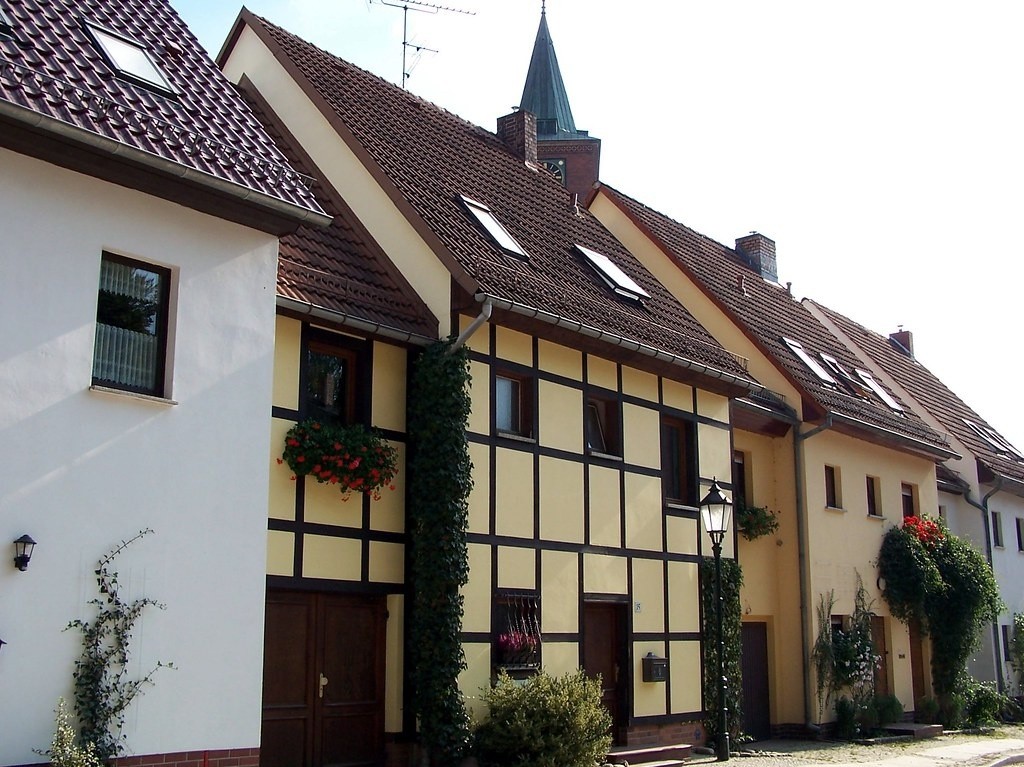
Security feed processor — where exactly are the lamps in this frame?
[13,535,38,571]
[877,575,886,591]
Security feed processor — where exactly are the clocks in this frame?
[540,158,568,187]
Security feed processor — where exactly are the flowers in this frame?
[497,628,537,652]
[277,417,400,502]
[736,506,781,542]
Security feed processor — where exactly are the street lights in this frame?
[700,472,736,761]
